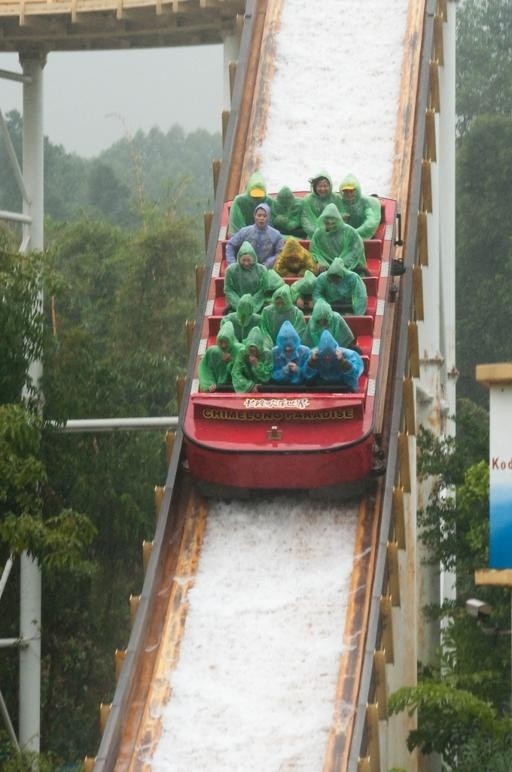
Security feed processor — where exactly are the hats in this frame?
[250,188,265,198]
[341,185,354,191]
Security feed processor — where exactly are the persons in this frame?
[197,321,242,392]
[270,185,307,240]
[312,257,367,316]
[289,269,317,315]
[220,293,265,343]
[228,171,275,236]
[224,202,284,269]
[300,169,351,241]
[222,240,266,316]
[302,297,355,349]
[260,268,285,314]
[272,236,317,277]
[308,203,367,278]
[302,330,365,392]
[230,326,272,393]
[259,285,306,345]
[339,173,381,240]
[270,320,311,385]
